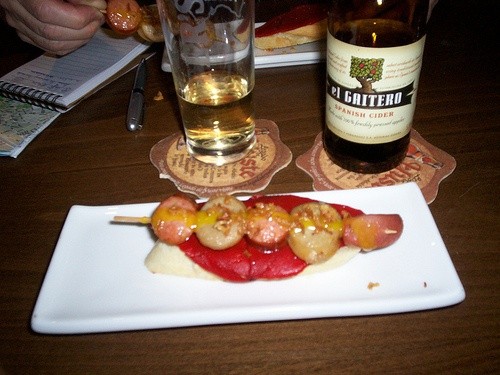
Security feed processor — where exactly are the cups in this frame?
[155,0,257,165]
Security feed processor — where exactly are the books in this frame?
[1,26,159,159]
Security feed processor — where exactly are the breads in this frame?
[234,16,251,43]
[254,2,328,50]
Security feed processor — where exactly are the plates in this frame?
[161,23,326,72]
[32,181,467,333]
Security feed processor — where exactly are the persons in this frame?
[0,0,109,56]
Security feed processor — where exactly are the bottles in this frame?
[322,0,429,175]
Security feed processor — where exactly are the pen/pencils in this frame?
[125,57,149,131]
